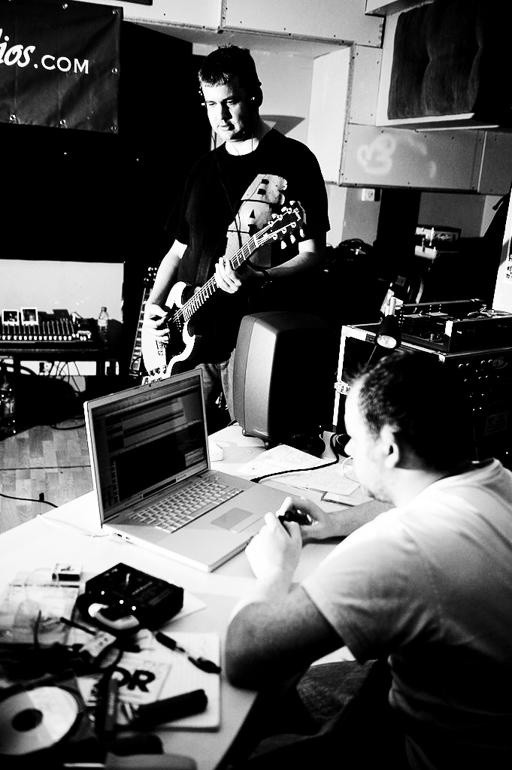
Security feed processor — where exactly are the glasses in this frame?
[199,94,258,108]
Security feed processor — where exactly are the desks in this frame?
[0,422,431,770]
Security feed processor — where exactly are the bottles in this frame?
[98,307,109,344]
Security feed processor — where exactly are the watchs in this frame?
[259,270,275,296]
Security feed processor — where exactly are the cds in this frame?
[0,686,81,756]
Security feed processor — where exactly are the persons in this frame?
[224,356,512,769]
[145,46,332,419]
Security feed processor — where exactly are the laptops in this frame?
[83,368,305,574]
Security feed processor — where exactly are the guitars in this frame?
[136,197,309,382]
